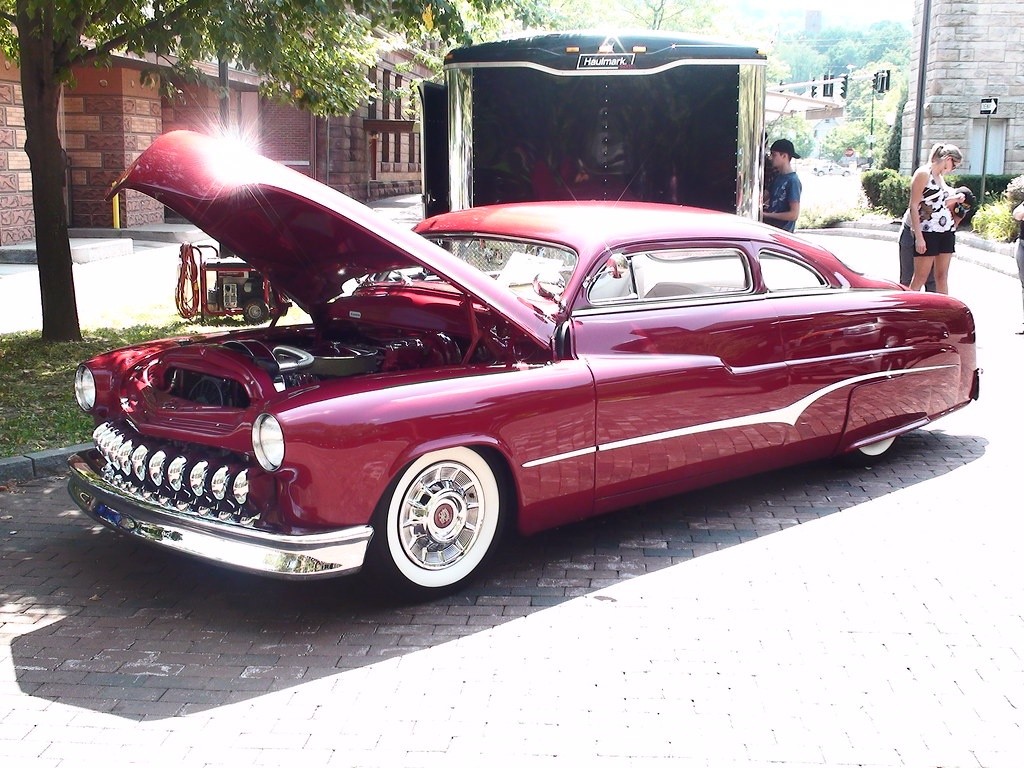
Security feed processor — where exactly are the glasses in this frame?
[952,159,956,171]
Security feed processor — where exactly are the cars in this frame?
[813,161,852,177]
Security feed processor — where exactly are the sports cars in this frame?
[60,129,986,605]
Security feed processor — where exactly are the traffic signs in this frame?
[981,99,999,115]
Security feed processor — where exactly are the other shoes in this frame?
[1015,324,1024,334]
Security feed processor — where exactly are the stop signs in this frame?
[845,148,854,157]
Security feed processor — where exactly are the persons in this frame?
[899,154,977,292]
[761,130,802,233]
[909,143,966,295]
[1013,199,1024,334]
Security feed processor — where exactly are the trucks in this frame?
[414,28,772,256]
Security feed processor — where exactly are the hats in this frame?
[769,138,801,159]
[954,186,974,218]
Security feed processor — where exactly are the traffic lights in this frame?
[839,76,848,99]
[871,71,884,93]
[810,77,819,98]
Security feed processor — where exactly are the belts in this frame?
[902,222,910,231]
